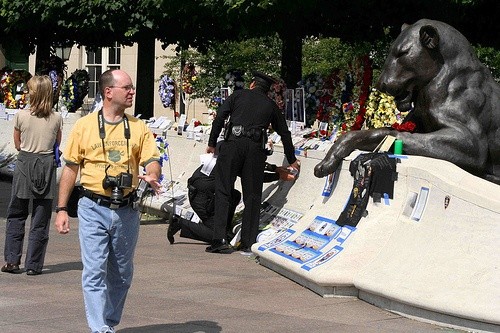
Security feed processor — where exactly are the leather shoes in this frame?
[236,244,250,252]
[206,238,229,253]
[166,213,181,245]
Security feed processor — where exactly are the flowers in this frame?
[0,57,91,113]
[159,73,175,109]
[179,63,198,93]
[194,121,207,127]
[311,63,416,138]
[268,76,287,111]
[209,97,226,117]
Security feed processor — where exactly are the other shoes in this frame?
[1,263,22,274]
[26,270,43,275]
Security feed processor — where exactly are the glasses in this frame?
[105,85,136,91]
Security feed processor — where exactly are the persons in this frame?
[167,141,290,250]
[54,69,162,333]
[204,70,301,253]
[0,74,63,276]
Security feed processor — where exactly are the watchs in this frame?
[56,206,67,212]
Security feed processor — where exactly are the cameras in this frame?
[102,172,133,205]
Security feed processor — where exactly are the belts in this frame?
[80,187,138,208]
